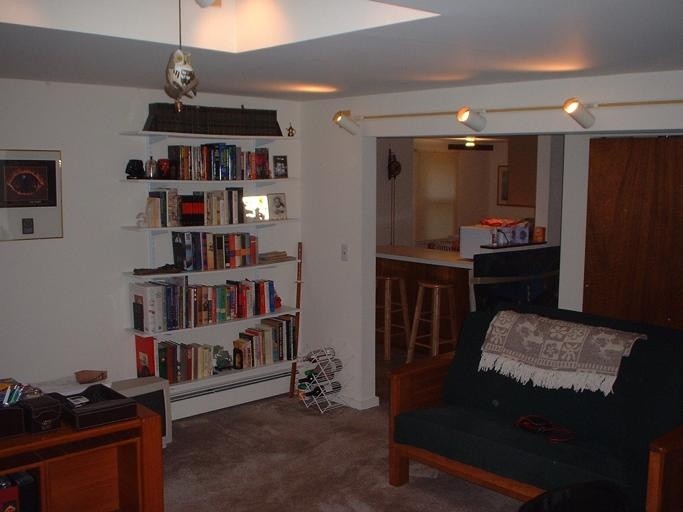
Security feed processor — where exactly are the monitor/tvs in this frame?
[112,376,173,449]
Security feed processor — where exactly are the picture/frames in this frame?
[0,148,63,241]
[496,164,508,207]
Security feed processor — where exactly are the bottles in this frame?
[297,347,342,398]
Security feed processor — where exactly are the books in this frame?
[134,142,298,385]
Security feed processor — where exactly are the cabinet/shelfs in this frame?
[295,342,347,415]
[507,136,535,208]
[0,400,165,512]
[119,130,302,423]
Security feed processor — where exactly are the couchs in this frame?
[380,299,683,512]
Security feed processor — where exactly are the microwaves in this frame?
[457,221,529,259]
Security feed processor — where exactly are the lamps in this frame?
[332,96,683,137]
[197,0,215,9]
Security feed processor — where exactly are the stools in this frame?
[376,268,411,365]
[406,282,459,364]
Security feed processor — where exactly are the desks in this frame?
[378,244,476,313]
